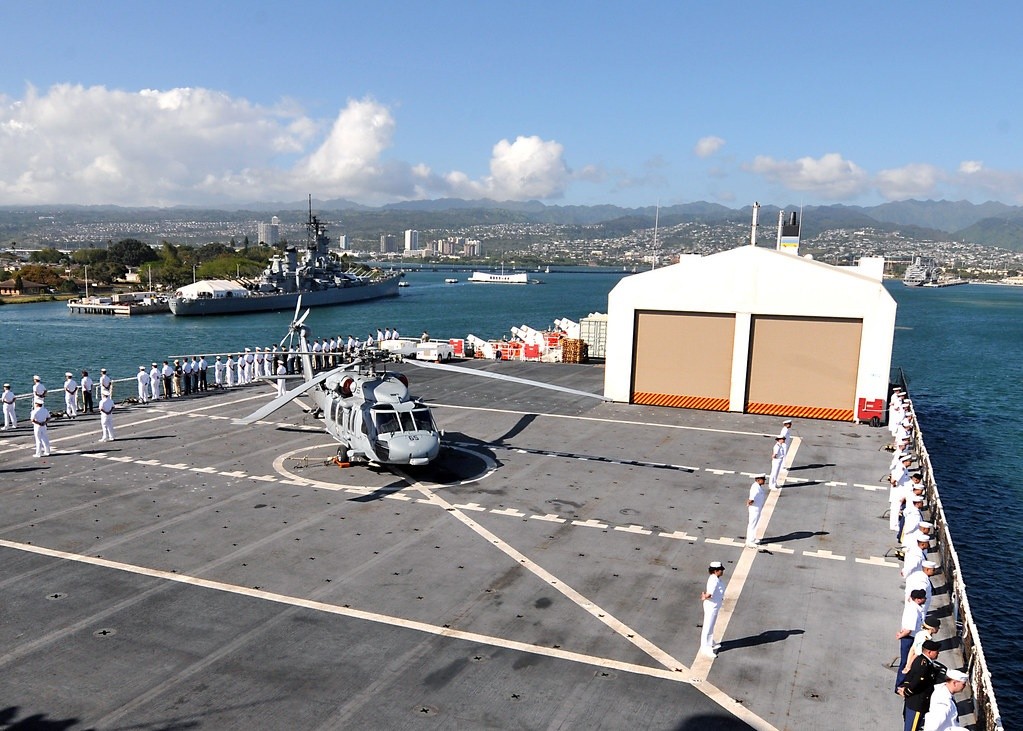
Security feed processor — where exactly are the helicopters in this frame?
[168,294,613,467]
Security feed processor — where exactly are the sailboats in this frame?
[545,266,551,273]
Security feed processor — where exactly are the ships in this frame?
[165,193,404,314]
[902,256,933,286]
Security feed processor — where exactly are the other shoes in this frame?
[746,539,760,548]
[12,425,16,428]
[43,452,50,455]
[98,439,106,442]
[32,454,41,457]
[108,437,114,440]
[701,644,721,658]
[1,427,9,430]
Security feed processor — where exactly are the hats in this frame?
[754,472,766,478]
[65,372,73,377]
[151,362,157,366]
[100,369,107,371]
[102,391,110,396]
[215,346,272,360]
[139,366,146,369]
[34,399,44,407]
[277,360,284,364]
[890,382,970,699]
[163,360,168,363]
[3,383,11,387]
[709,561,722,567]
[776,434,785,439]
[783,419,792,424]
[33,375,41,380]
[355,337,359,339]
[174,360,179,363]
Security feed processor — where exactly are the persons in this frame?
[80,371,93,412]
[30,400,50,457]
[100,369,111,400]
[888,386,968,731]
[64,371,77,416]
[149,362,160,401]
[161,360,173,398]
[746,474,766,547]
[700,561,725,657]
[98,391,114,442]
[421,331,429,342]
[173,327,399,397]
[780,420,792,472]
[274,360,286,398]
[137,366,149,404]
[1,383,17,430]
[768,435,786,490]
[32,375,46,409]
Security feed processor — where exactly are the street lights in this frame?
[193,262,202,283]
[147,264,151,291]
[83,265,90,298]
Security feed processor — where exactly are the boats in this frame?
[398,281,409,287]
[445,279,458,283]
[468,258,540,285]
